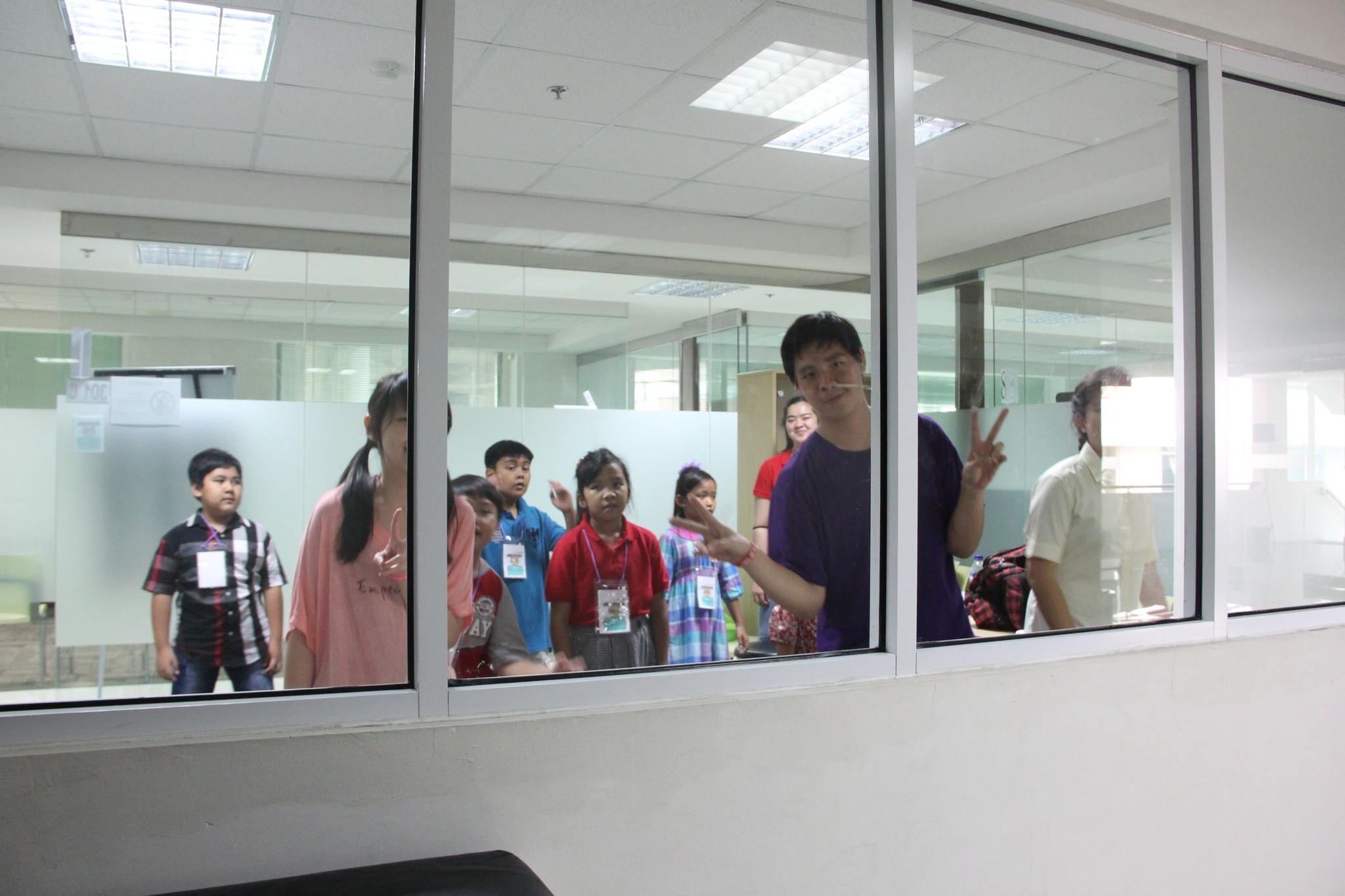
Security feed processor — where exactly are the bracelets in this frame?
[731,543,758,571]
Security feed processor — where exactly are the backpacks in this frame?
[963,545,1031,631]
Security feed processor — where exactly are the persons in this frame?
[752,393,818,660]
[547,446,672,676]
[483,438,575,676]
[139,447,287,701]
[447,473,584,683]
[767,600,823,661]
[278,371,476,692]
[1014,366,1177,634]
[667,310,1015,654]
[658,462,752,666]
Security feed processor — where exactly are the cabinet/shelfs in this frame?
[737,369,872,638]
